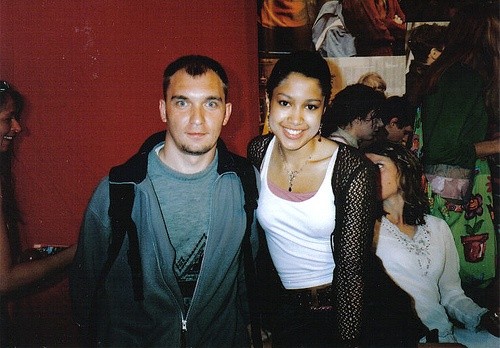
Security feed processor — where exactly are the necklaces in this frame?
[278,136,318,193]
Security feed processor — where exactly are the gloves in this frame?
[483,311,500,338]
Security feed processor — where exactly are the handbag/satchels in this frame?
[428,164,471,203]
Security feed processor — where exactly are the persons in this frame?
[1,80,78,348]
[382,97,416,145]
[358,140,499,348]
[321,83,387,150]
[74,56,261,348]
[246,49,381,348]
[258,0,447,94]
[403,0,498,333]
